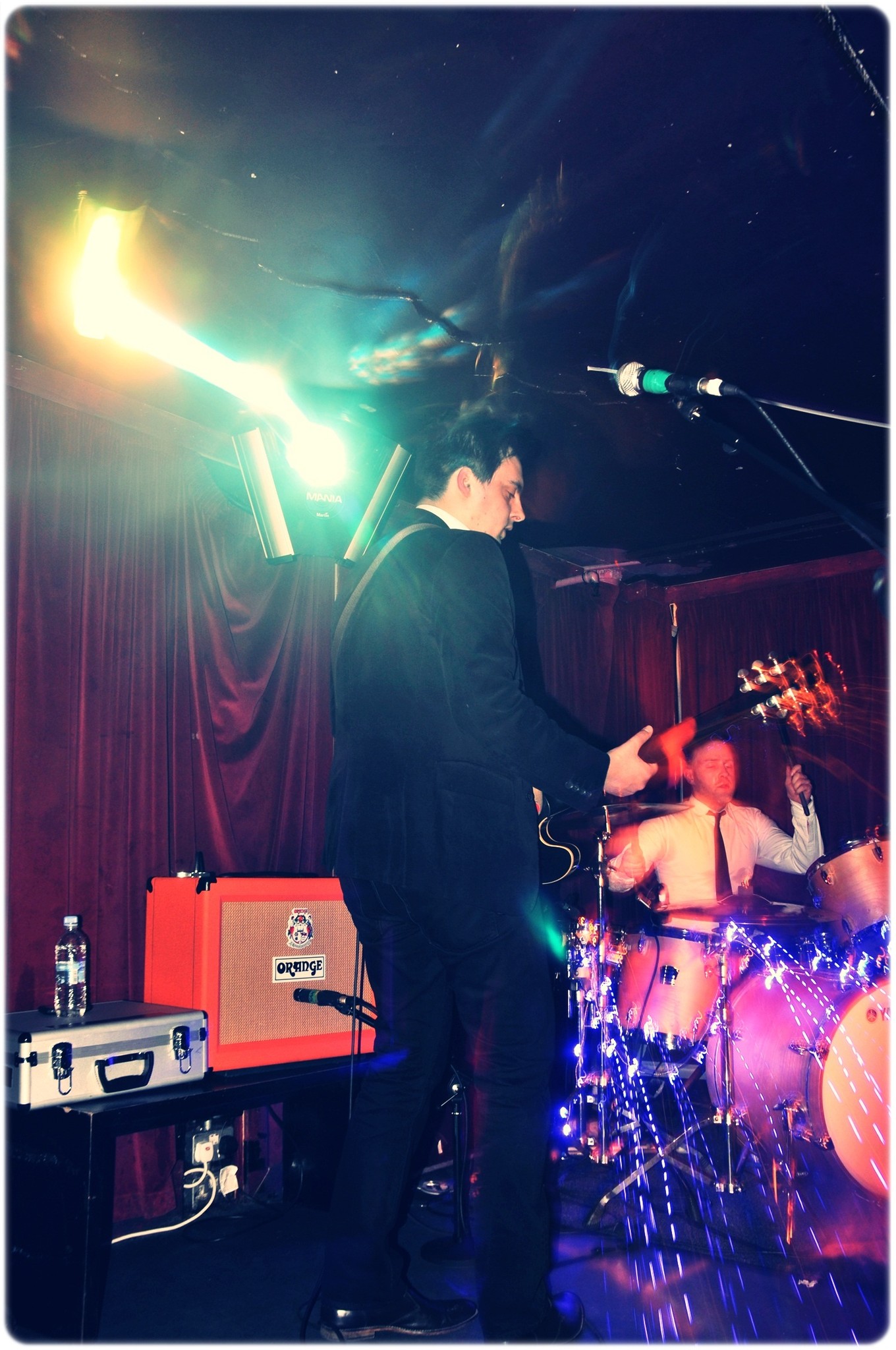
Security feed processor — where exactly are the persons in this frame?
[321,418,658,1344]
[607,735,826,936]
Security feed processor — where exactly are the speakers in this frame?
[143,878,378,1072]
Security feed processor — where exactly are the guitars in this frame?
[532,649,845,885]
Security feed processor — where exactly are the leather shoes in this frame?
[320,1286,478,1342]
[484,1290,586,1343]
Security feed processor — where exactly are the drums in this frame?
[803,834,891,948]
[707,962,890,1201]
[607,926,755,1052]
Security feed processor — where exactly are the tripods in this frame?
[421,813,827,1238]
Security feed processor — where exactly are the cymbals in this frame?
[561,802,694,843]
[657,892,837,926]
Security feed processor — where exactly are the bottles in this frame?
[54,916,87,1017]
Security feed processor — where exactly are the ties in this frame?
[706,811,734,900]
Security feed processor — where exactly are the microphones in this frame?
[616,360,742,398]
[293,989,361,1008]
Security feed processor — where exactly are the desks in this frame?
[7,1040,406,1343]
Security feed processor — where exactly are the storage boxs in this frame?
[8,869,378,1111]
